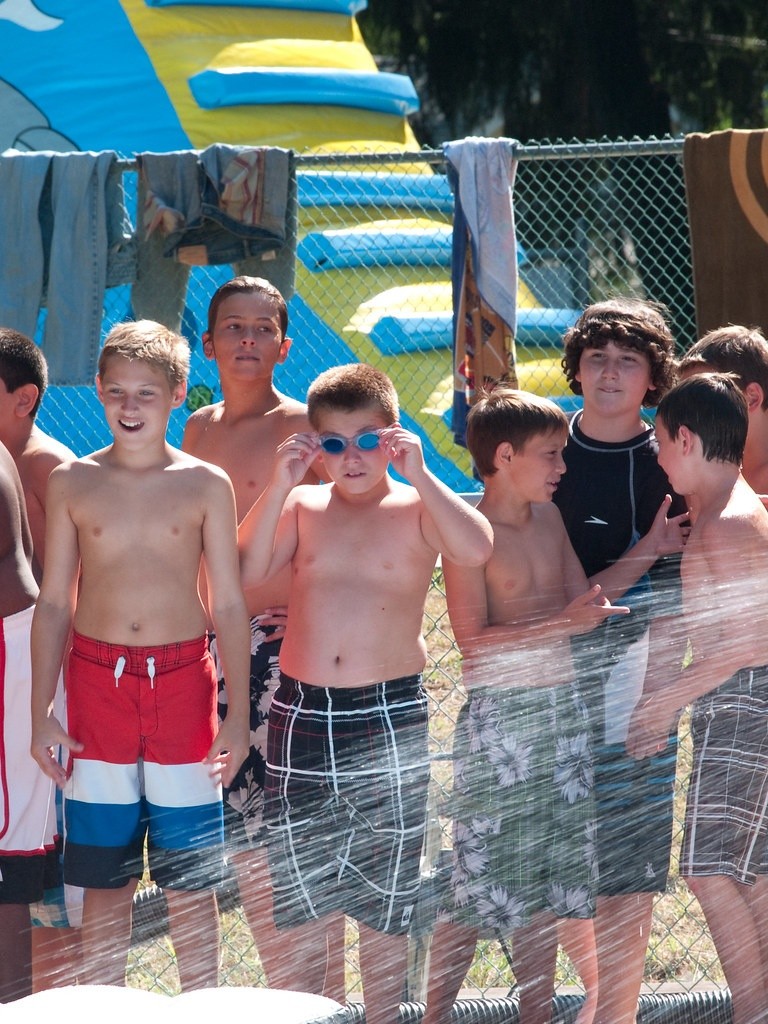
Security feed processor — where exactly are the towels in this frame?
[442,137,520,487]
[683,127,768,351]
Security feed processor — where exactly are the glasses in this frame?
[318,431,380,454]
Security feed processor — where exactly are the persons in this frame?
[29,318,252,992]
[420,389,693,1024]
[238,364,493,1024]
[1,276,767,1024]
